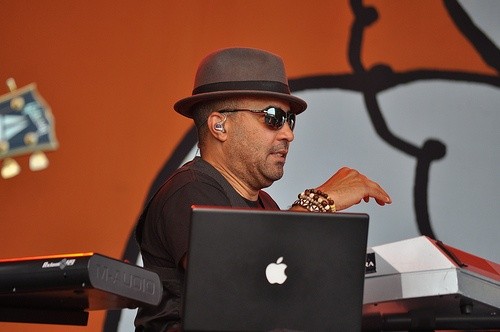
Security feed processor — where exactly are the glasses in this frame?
[217,106,295,132]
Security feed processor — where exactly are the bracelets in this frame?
[292,187,337,213]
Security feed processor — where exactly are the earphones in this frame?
[214,122,225,133]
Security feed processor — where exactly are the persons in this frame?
[134,48,392,332]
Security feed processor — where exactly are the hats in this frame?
[173,48,307,119]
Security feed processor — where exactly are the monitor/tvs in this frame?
[181,204,370,332]
